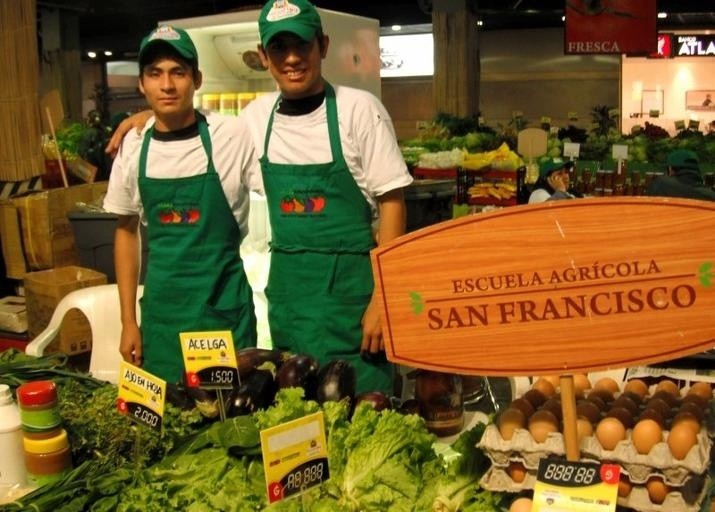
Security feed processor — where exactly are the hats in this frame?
[540,158,574,179]
[139,24,198,58]
[665,150,699,167]
[259,1,322,48]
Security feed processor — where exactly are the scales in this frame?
[404,179,457,235]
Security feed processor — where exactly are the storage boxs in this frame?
[21,266,110,359]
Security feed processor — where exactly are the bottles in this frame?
[0,384,27,487]
[581,167,665,194]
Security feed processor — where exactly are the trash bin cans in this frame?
[66,211,119,284]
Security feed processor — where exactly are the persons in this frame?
[104,0,416,402]
[527,152,583,206]
[644,148,715,202]
[102,22,269,390]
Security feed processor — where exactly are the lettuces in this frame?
[122,385,503,512]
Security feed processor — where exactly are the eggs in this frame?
[496,372,713,512]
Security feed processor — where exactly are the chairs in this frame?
[24,285,145,386]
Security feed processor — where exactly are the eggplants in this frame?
[626,136,649,161]
[165,347,422,431]
[539,135,571,163]
